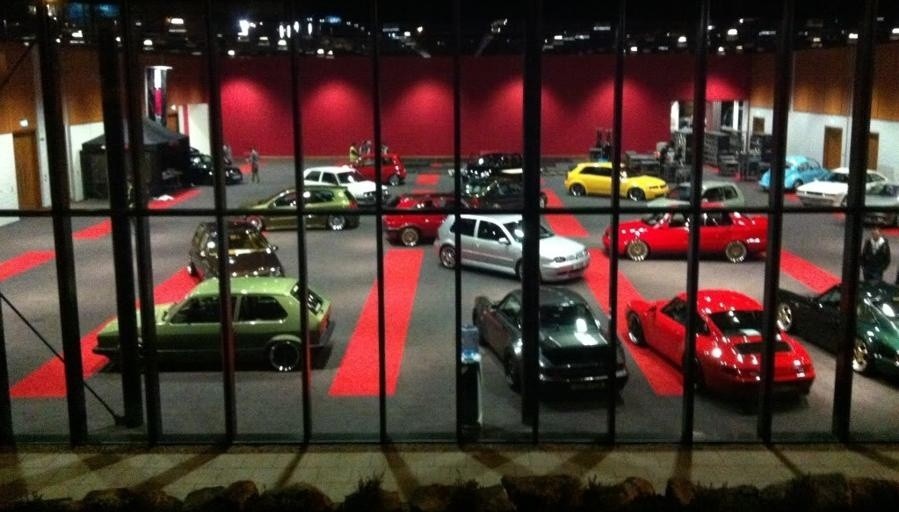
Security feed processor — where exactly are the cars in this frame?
[303,165,388,206]
[186,222,286,282]
[560,159,671,203]
[796,165,892,211]
[598,211,770,263]
[89,276,336,374]
[233,183,362,233]
[449,175,549,212]
[461,152,523,182]
[644,177,746,216]
[188,151,243,187]
[863,181,899,228]
[386,190,478,247]
[757,154,831,192]
[336,152,408,188]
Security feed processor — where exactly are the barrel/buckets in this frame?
[460,324,480,365]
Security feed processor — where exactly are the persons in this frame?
[381,144,391,160]
[245,148,260,183]
[349,143,361,161]
[861,227,891,281]
[223,144,233,165]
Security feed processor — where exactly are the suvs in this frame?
[432,208,597,283]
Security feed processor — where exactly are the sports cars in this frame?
[775,273,899,391]
[458,284,631,399]
[620,282,819,401]
